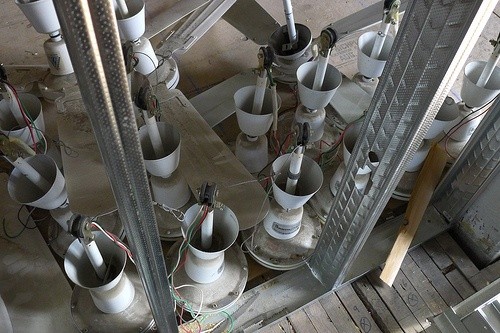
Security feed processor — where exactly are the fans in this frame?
[0,0,500,333]
